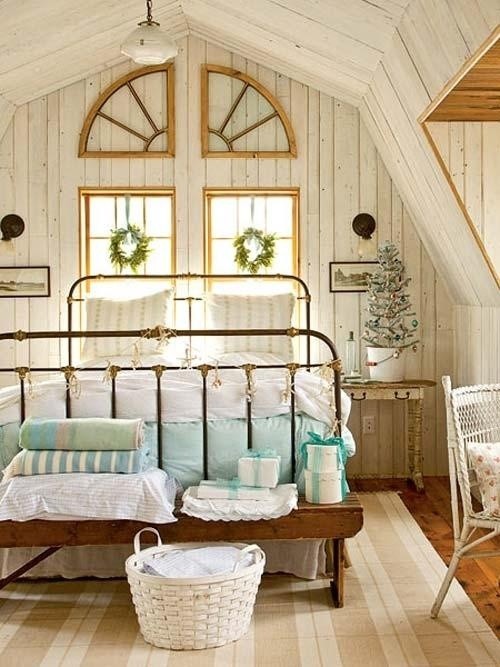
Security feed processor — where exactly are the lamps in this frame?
[119,0,179,65]
[352,212,379,262]
[0,211,25,267]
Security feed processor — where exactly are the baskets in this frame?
[125,527,267,650]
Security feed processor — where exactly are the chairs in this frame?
[431,375,500,620]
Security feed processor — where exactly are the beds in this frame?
[0,271,341,579]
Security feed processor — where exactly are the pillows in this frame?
[0,466,176,524]
[82,292,296,365]
[468,440,498,522]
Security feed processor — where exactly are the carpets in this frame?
[0,489,500,666]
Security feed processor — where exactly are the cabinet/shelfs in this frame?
[339,378,438,494]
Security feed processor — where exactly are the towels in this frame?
[3,417,147,476]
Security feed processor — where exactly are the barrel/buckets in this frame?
[364,346,406,381]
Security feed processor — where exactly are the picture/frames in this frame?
[330,260,383,292]
[0,266,51,298]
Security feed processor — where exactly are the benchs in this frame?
[0,492,365,607]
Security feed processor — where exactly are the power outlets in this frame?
[363,415,375,435]
